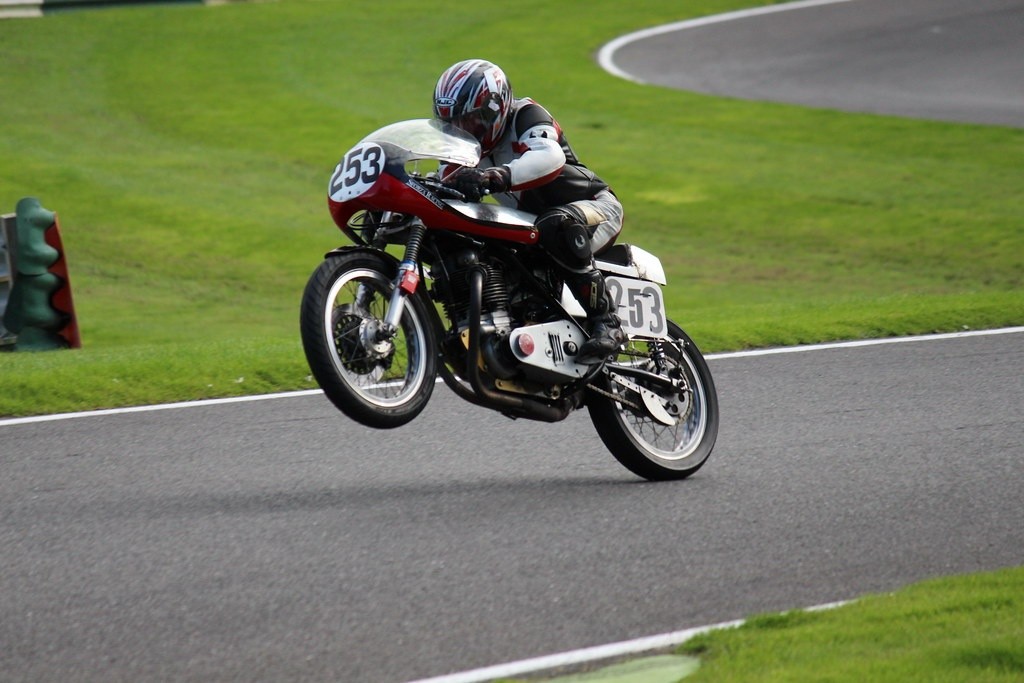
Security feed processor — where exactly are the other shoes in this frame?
[578,323,630,360]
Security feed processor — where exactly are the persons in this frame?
[432,57,629,360]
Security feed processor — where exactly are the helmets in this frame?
[432,58,512,161]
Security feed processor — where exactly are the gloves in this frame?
[448,164,512,203]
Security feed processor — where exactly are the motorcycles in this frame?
[298,120,719,479]
